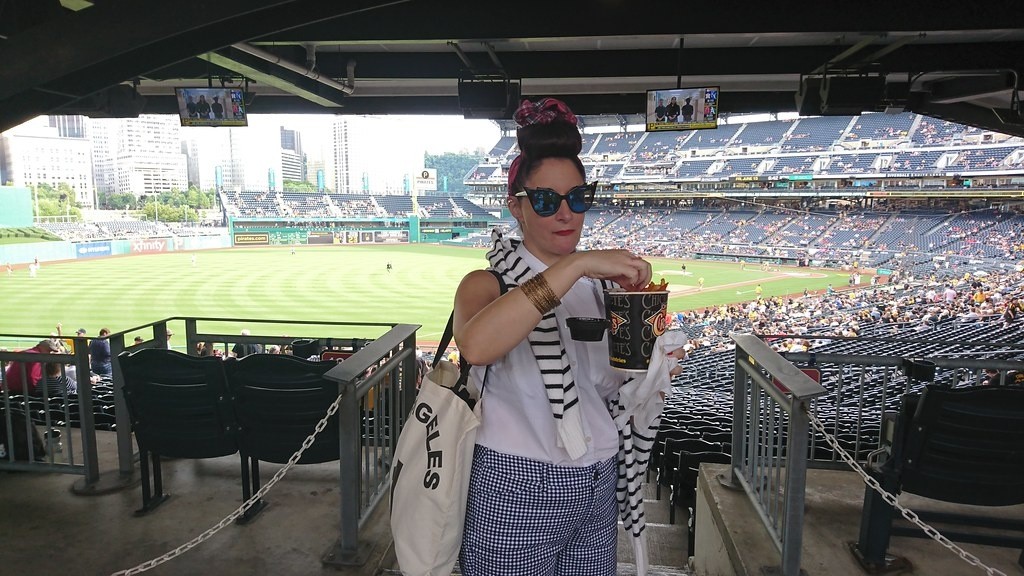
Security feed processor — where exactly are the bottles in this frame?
[44,428,64,462]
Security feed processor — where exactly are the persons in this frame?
[186,95,243,119]
[453,99,686,576]
[653,97,715,125]
[575,121,1024,387]
[227,190,518,273]
[4,219,222,278]
[0,322,460,420]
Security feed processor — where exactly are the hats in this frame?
[40,340,58,352]
[167,329,174,336]
[135,336,143,341]
[76,329,86,334]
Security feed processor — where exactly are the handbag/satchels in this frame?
[388,359,484,576]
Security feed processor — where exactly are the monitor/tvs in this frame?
[646,86,720,132]
[174,86,248,127]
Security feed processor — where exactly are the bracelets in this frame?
[520,272,563,316]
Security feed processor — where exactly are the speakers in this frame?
[459,81,521,120]
[44,84,147,118]
[795,76,889,116]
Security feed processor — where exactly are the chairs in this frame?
[0,384,408,445]
[117,351,362,523]
[850,381,1024,576]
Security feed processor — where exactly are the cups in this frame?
[604,291,669,373]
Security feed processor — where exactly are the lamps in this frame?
[795,61,888,116]
[456,69,522,119]
[82,78,147,119]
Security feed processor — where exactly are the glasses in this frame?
[515,181,598,216]
